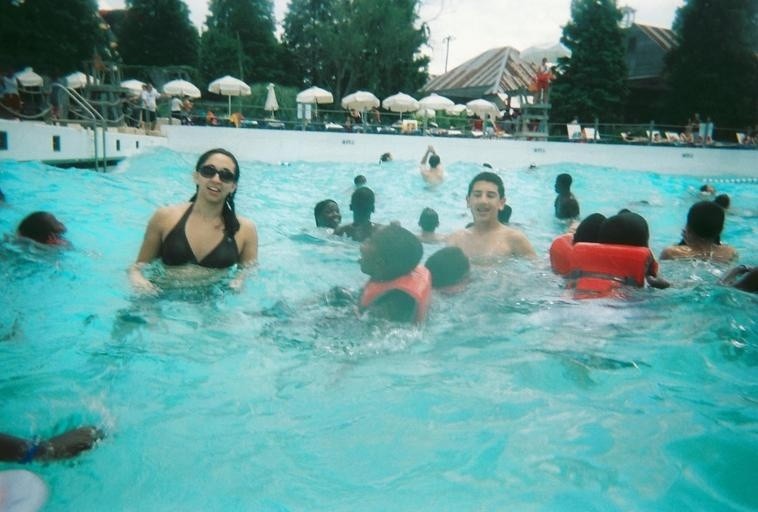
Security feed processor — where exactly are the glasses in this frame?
[199,164,236,185]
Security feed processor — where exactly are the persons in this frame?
[553,173,580,219]
[14,211,68,248]
[127,84,146,123]
[659,200,737,264]
[424,245,473,299]
[369,105,383,129]
[711,190,733,209]
[182,95,194,125]
[169,94,183,123]
[1,68,21,114]
[498,206,521,226]
[87,147,258,372]
[142,83,171,133]
[528,160,538,169]
[341,231,341,232]
[349,175,375,228]
[378,151,395,165]
[448,172,538,263]
[619,116,758,148]
[415,207,449,243]
[572,213,758,307]
[313,198,342,235]
[419,144,444,191]
[546,212,607,288]
[323,225,431,324]
[349,106,363,129]
[1,424,106,464]
[482,114,496,137]
[695,182,716,195]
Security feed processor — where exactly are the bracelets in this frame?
[18,434,42,466]
